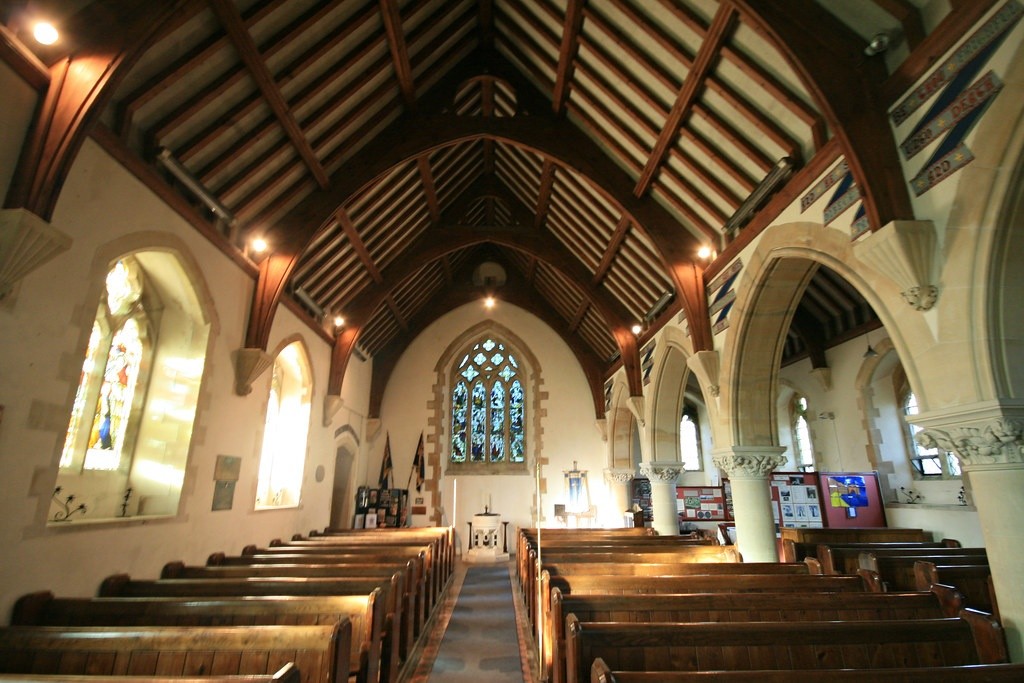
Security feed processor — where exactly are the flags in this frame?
[412,434,425,492]
[378,435,392,485]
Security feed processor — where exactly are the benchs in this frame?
[517,523,1024,683]
[0,526,455,683]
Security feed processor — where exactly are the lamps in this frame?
[609,350,620,363]
[481,276,497,312]
[644,290,673,322]
[156,146,238,227]
[295,285,327,318]
[723,157,793,235]
[845,33,891,79]
[863,332,879,357]
